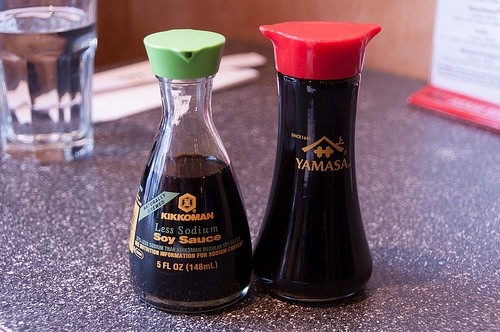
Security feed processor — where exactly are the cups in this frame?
[0,0,98,164]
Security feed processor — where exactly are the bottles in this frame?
[254,21,381,305]
[129,28,255,315]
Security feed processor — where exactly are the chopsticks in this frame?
[92,51,266,92]
